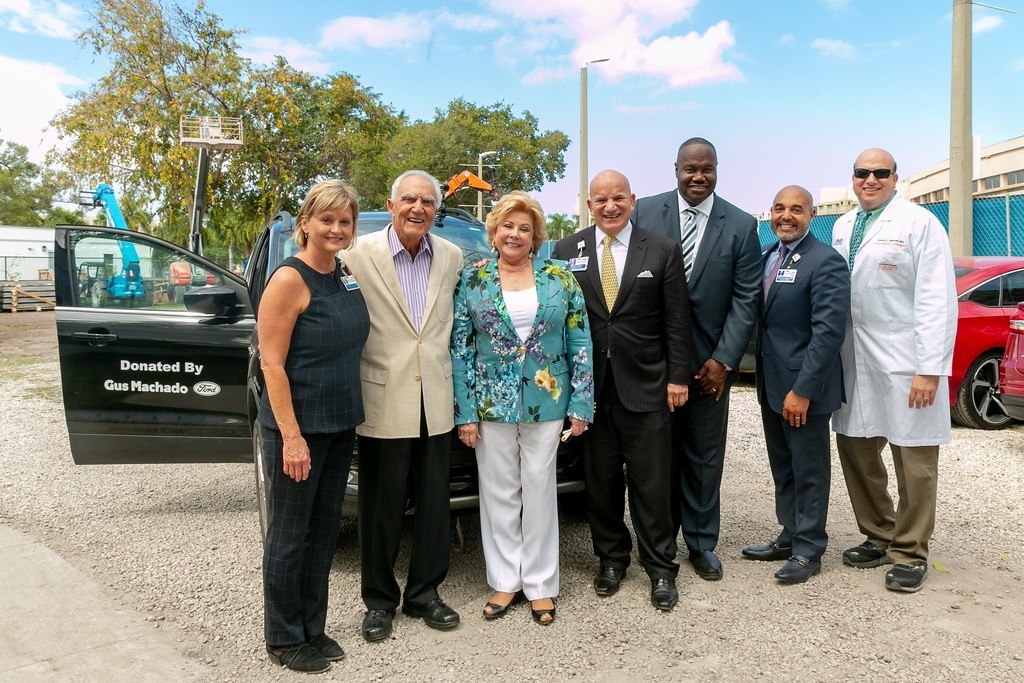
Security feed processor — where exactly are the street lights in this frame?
[478,151,496,221]
[579,58,610,231]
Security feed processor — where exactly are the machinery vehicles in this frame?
[167,114,246,303]
[78,186,148,308]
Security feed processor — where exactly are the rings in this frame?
[795,416,800,418]
[924,397,930,400]
[585,426,588,431]
[459,437,464,439]
[572,422,577,425]
[712,387,716,392]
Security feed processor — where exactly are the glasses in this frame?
[854,169,894,178]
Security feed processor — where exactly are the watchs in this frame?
[724,364,732,372]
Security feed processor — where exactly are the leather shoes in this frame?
[689,549,723,580]
[651,579,678,610]
[742,540,792,561]
[774,555,822,582]
[362,609,396,642]
[595,565,626,596]
[402,599,460,629]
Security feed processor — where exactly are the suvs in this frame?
[53,206,628,548]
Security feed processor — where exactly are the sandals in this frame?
[483,590,528,619]
[530,598,555,626]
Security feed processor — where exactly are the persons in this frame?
[832,149,958,593]
[254,180,371,674]
[631,137,763,581]
[742,185,849,585]
[551,171,691,612]
[339,170,465,642]
[450,190,596,626]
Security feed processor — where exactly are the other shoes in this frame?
[266,633,345,673]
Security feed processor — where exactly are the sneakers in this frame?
[843,541,894,567]
[885,561,928,592]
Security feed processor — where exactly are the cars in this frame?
[948,257,1023,431]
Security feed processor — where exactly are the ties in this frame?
[762,245,789,305]
[849,210,872,278]
[681,208,698,283]
[602,235,619,314]
